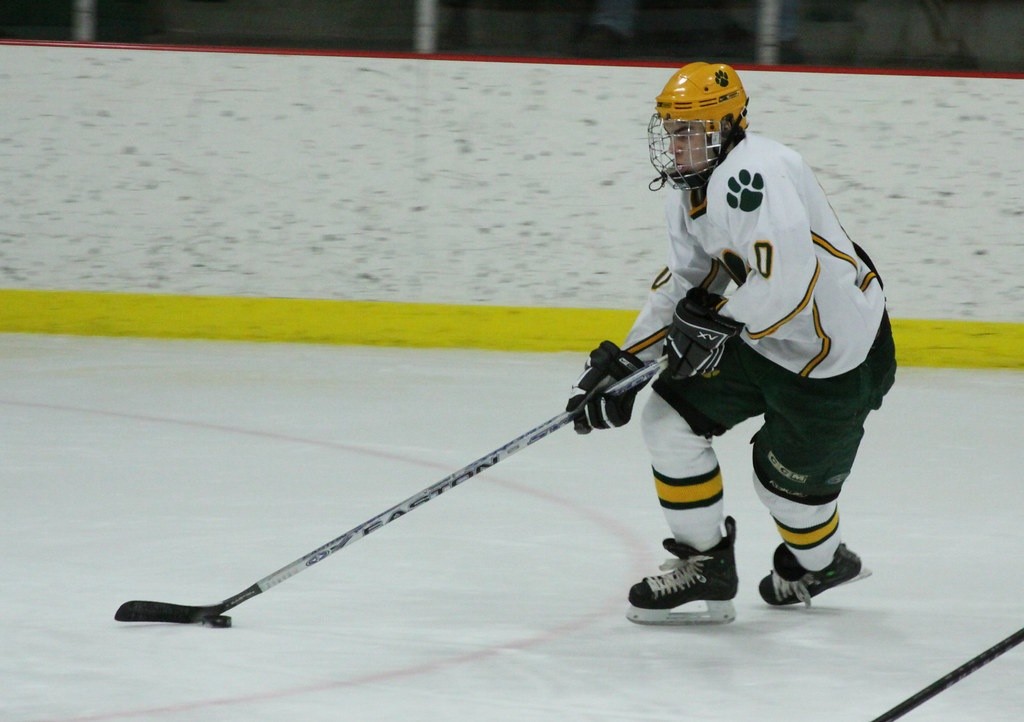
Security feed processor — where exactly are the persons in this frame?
[565,62,896,627]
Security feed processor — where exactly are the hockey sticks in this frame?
[110,353,673,626]
[870,626,1024,721]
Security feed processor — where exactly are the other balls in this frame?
[201,615,232,629]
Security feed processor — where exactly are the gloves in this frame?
[661,286,746,380]
[567,341,645,435]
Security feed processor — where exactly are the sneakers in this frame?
[626,516,738,624]
[760,543,871,608]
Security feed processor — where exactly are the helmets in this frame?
[648,63,750,191]
